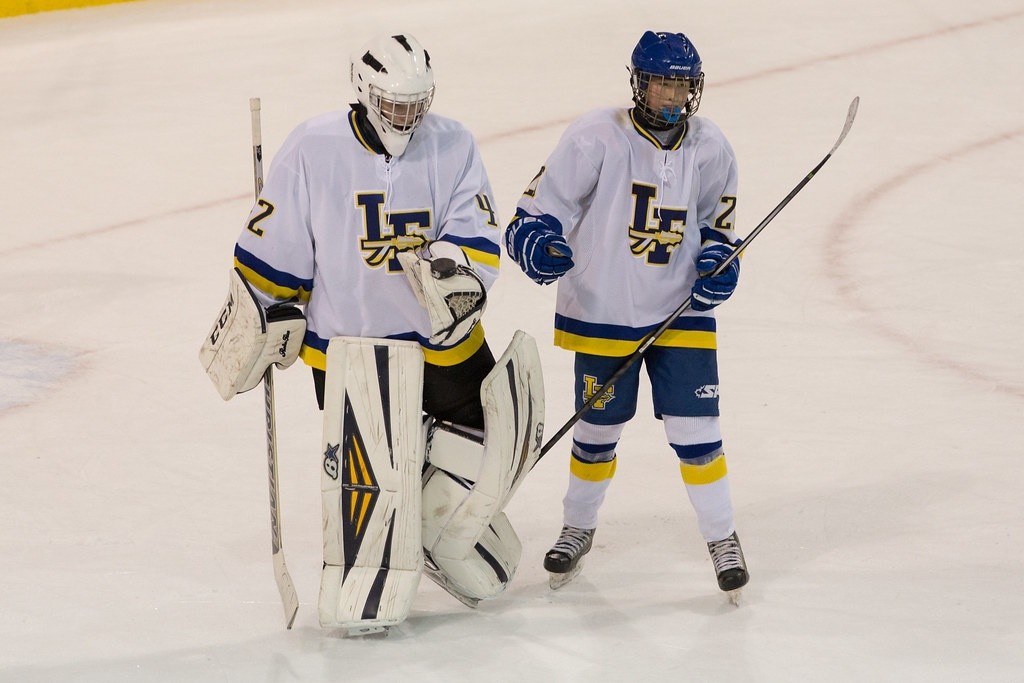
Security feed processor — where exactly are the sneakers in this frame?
[543,523,596,590]
[707,530,748,608]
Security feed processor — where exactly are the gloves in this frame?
[507,218,575,285]
[691,246,740,311]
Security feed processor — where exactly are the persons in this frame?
[502,31,750,608]
[199,31,546,636]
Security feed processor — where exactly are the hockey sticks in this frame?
[527,96,861,471]
[249,97,300,630]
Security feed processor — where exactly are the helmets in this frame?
[628,30,705,131]
[355,33,436,158]
